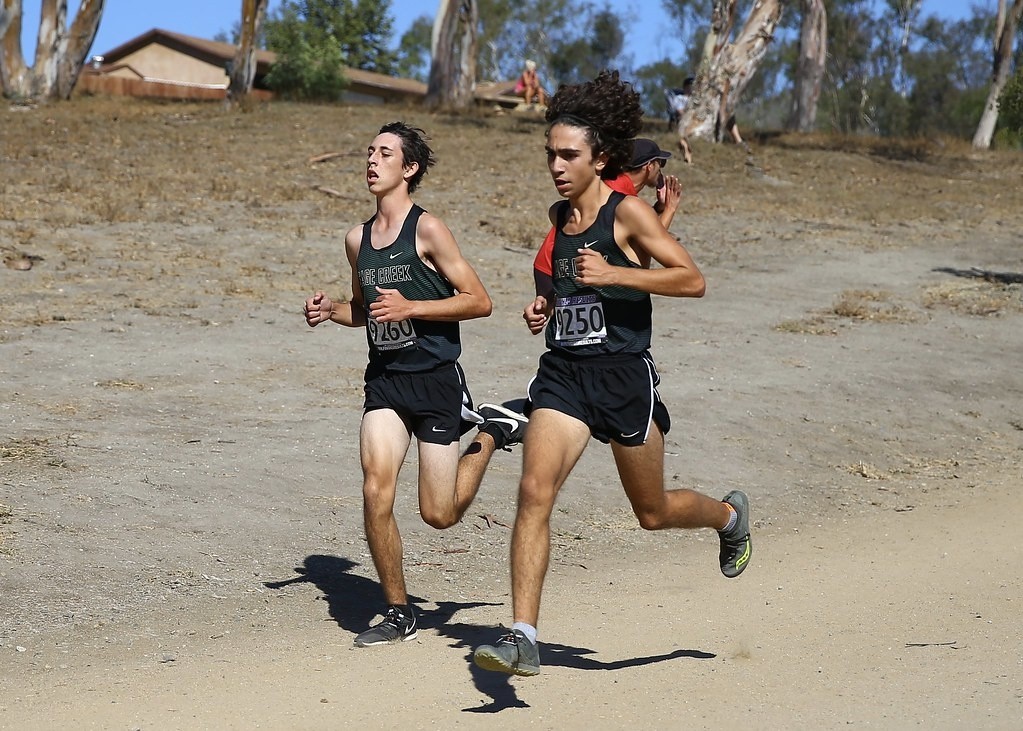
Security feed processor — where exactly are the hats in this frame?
[619,138,671,168]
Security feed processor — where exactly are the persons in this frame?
[474,70,752,676]
[304,121,530,648]
[533,136,682,348]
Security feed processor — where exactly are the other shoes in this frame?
[735,141,753,154]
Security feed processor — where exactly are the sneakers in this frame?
[478,403,530,451]
[720,490,753,576]
[353,606,419,647]
[474,628,541,676]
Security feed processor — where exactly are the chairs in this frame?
[664,87,687,130]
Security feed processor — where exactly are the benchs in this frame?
[474,91,541,107]
